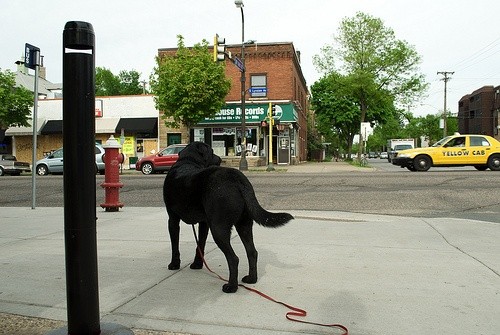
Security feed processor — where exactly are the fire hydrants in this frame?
[99,135,125,213]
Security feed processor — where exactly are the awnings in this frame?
[5,118,45,136]
[94,118,120,133]
[115,115,159,133]
[42,120,67,134]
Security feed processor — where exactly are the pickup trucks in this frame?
[0,154,30,176]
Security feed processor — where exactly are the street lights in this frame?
[233,0,248,171]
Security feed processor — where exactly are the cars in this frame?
[379,151,390,158]
[361,151,379,158]
[396,132,500,173]
[36,141,105,177]
[135,143,191,174]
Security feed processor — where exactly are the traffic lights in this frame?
[213,33,231,63]
[269,103,275,116]
[261,120,267,127]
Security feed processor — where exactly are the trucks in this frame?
[387,138,415,165]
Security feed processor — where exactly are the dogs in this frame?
[162,140,295,294]
